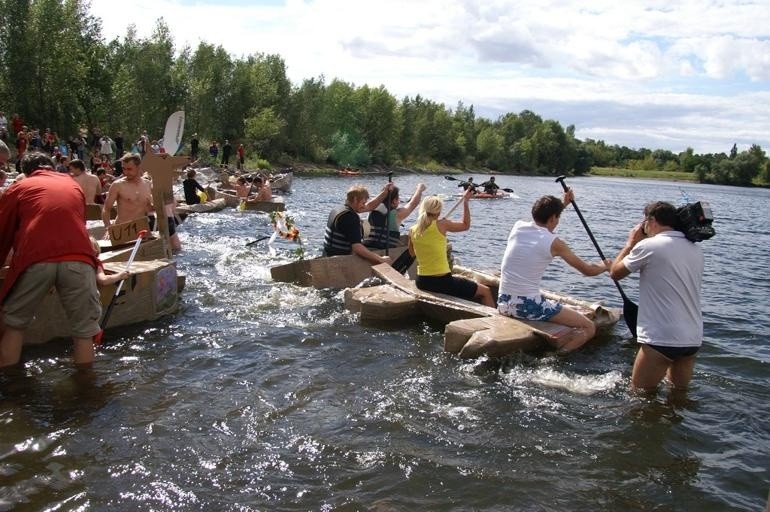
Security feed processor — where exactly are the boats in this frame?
[341,253,620,368]
[0,170,296,345]
[463,191,508,199]
[267,238,454,291]
[339,165,361,177]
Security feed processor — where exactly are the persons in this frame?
[610,200,704,391]
[408,186,495,309]
[497,186,612,355]
[323,182,394,265]
[190,134,244,170]
[183,170,205,204]
[219,169,270,202]
[341,163,360,172]
[480,177,500,194]
[1,111,181,368]
[458,178,479,195]
[362,184,426,249]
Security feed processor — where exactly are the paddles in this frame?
[152,110,185,233]
[555,175,639,339]
[445,176,512,193]
[238,178,253,210]
[393,186,472,275]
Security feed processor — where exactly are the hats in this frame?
[368,204,388,227]
